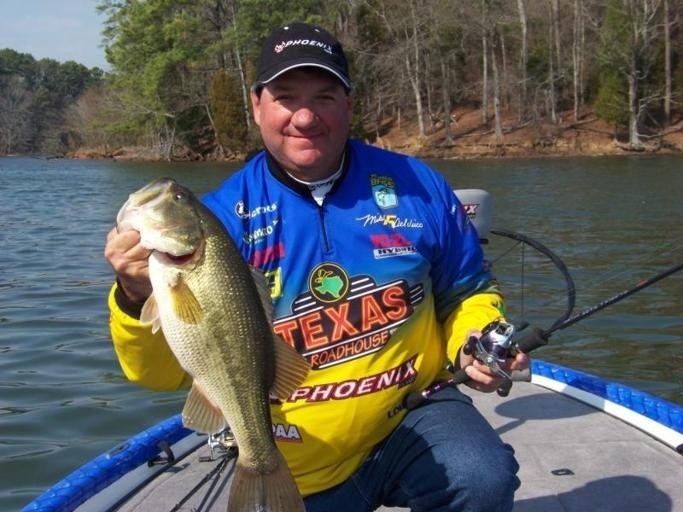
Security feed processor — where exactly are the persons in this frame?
[102,20,532,512]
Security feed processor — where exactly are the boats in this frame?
[24,356,683,508]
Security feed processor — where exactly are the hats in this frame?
[254,21,351,95]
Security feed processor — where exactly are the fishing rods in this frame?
[170,430,240,512]
[403,262,683,408]
